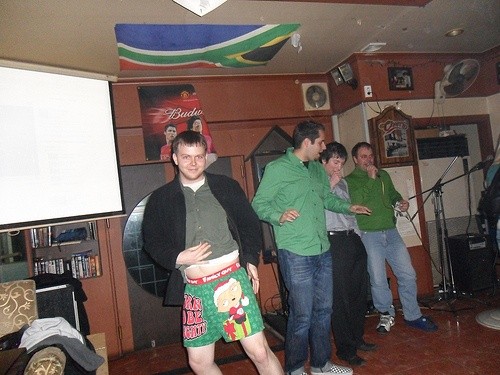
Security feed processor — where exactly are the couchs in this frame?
[0,280,66,375]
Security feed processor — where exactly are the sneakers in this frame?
[376,313,396,334]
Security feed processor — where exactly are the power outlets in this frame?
[364,86,372,97]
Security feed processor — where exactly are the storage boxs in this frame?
[87,333,109,375]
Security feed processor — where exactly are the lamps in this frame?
[331,63,356,90]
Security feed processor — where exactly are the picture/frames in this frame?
[387,66,414,90]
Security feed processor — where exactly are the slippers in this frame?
[404,315,439,332]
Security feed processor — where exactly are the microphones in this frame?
[367,162,381,178]
[476,154,494,167]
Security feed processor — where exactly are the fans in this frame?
[435,58,480,105]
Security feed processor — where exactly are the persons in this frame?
[393,72,410,87]
[251,120,371,375]
[188,115,216,154]
[318,141,376,366]
[161,124,177,158]
[344,142,437,334]
[142,131,284,375]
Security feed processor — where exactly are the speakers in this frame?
[444,232,494,291]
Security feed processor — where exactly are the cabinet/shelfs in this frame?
[31,221,102,280]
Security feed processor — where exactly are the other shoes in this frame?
[343,354,367,366]
[298,372,309,375]
[357,341,377,352]
[311,363,353,375]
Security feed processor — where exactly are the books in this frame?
[30,226,51,248]
[33,250,101,279]
[52,240,81,246]
[87,222,96,240]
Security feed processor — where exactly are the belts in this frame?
[328,229,355,237]
